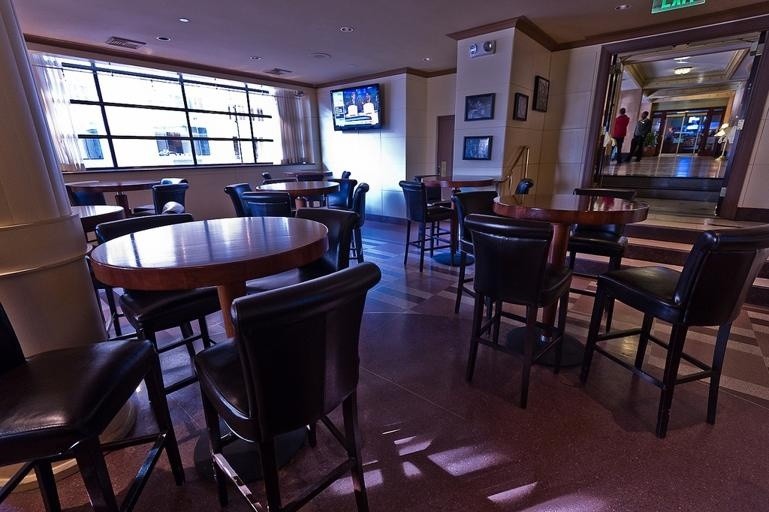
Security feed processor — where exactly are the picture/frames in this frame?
[463,92,496,120]
[531,72,551,116]
[461,135,493,161]
[512,92,529,123]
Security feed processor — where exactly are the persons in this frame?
[359,92,375,114]
[628,109,650,165]
[345,91,359,115]
[611,105,626,165]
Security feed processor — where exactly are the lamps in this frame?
[468,37,497,59]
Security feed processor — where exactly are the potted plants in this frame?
[642,132,656,154]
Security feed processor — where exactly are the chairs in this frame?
[580,223,769,438]
[399,172,457,274]
[67,164,370,411]
[451,188,648,368]
[1,300,184,511]
[464,213,572,411]
[194,259,382,511]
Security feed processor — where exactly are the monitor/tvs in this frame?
[330,84,384,130]
